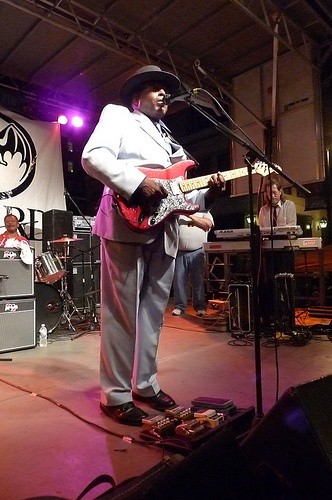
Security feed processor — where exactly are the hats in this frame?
[119,65,181,106]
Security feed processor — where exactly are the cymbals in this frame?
[50,238,84,243]
[60,257,71,260]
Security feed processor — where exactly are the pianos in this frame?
[203,225,322,324]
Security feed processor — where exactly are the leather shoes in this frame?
[132,389,176,412]
[100,400,148,425]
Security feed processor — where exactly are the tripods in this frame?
[48,243,101,334]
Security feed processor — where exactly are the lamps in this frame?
[317,217,327,231]
[246,214,256,224]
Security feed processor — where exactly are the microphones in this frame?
[162,88,201,104]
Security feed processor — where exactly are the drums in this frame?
[35,251,67,285]
[34,282,64,336]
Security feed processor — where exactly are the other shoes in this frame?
[171,308,183,316]
[196,310,206,316]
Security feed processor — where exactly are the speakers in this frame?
[42,210,72,256]
[0,247,35,297]
[70,232,100,264]
[140,375,332,500]
[70,265,100,309]
[0,298,36,352]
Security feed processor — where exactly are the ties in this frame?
[272,205,278,227]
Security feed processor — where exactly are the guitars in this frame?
[112,161,284,231]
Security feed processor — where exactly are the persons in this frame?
[258,179,297,326]
[171,211,214,316]
[81,68,225,426]
[0,214,30,248]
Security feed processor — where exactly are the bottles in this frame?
[39,324,47,347]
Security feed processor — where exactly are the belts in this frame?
[179,247,202,253]
